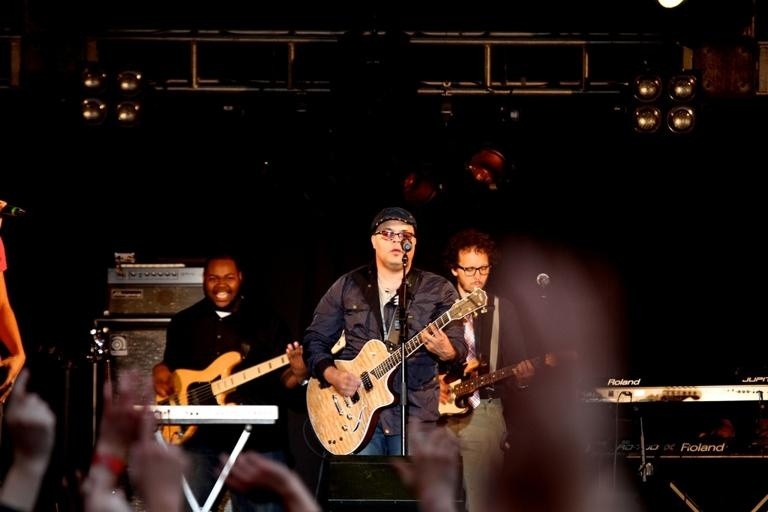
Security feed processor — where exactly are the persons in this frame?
[0,249,639,512]
[303,203,468,456]
[0,199,27,404]
[417,226,537,510]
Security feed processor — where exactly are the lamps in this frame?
[396,72,519,205]
[76,53,151,134]
[619,42,708,139]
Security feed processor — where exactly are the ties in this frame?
[463,313,480,409]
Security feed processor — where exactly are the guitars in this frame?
[436,353,559,414]
[306,288,487,457]
[156,331,346,445]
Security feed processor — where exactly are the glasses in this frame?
[457,265,491,276]
[374,228,417,240]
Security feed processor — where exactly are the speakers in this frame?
[93,318,171,406]
[315,454,467,512]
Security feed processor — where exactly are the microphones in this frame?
[535,273,551,299]
[399,238,412,251]
[0,205,26,217]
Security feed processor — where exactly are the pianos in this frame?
[130,403,278,426]
[576,383,768,405]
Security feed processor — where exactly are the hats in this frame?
[368,207,417,234]
